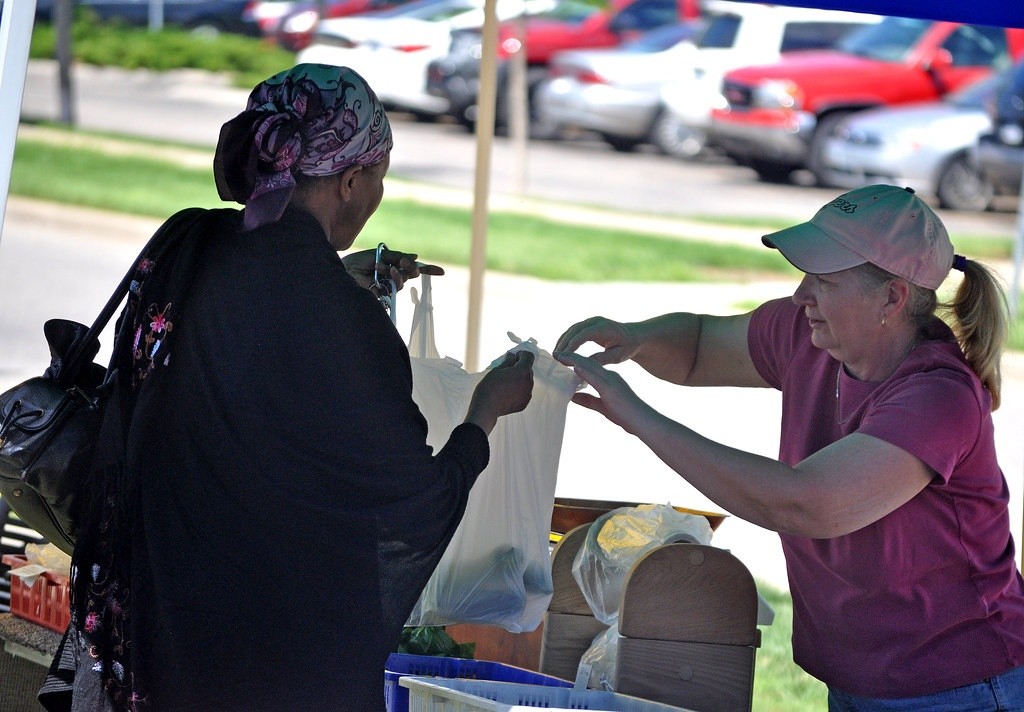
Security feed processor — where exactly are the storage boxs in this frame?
[2,553,71,634]
[386,653,696,711]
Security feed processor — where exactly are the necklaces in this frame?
[835,339,918,424]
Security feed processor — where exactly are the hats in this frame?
[762,184,955,292]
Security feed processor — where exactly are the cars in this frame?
[703,16,1024,185]
[810,60,1024,212]
[93,0,895,163]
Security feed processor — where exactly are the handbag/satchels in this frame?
[405,260,589,634]
[0,207,202,559]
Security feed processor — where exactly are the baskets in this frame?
[382,652,696,711]
[2,553,73,635]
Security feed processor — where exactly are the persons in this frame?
[72,62,536,712]
[552,184,1023,712]
